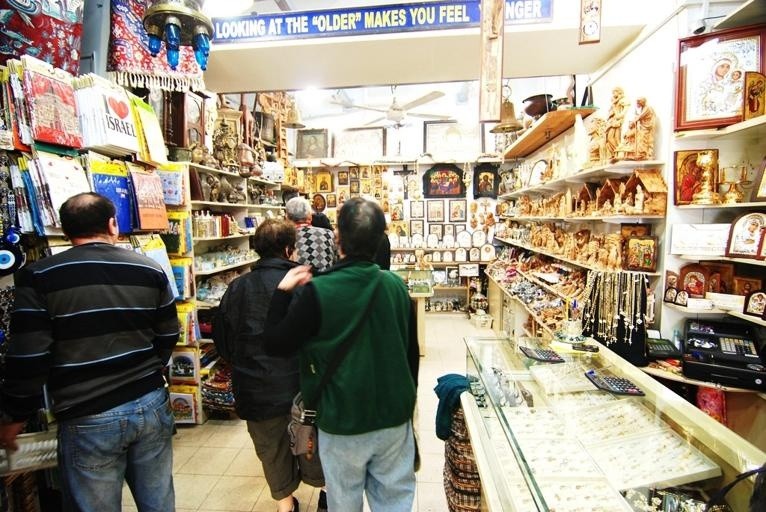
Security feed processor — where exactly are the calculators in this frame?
[519,346,565,362]
[584,373,645,397]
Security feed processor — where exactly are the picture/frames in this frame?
[300,162,502,286]
[295,129,327,158]
[330,125,387,158]
[422,119,459,154]
[663,25,765,317]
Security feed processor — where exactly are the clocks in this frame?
[162,85,212,149]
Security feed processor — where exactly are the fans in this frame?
[328,84,452,128]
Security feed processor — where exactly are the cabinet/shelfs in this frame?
[458,334,766,512]
[390,247,471,315]
[637,0,766,395]
[158,161,298,429]
[483,107,665,342]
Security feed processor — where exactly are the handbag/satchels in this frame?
[287,391,317,456]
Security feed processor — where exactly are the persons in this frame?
[734,218,760,254]
[751,294,765,313]
[581,116,606,166]
[633,96,656,160]
[281,188,419,395]
[262,197,417,512]
[211,217,328,511]
[739,283,751,296]
[678,155,705,201]
[687,274,704,297]
[622,120,637,159]
[604,87,624,160]
[304,136,323,158]
[478,175,493,194]
[694,53,742,114]
[0,192,180,512]
